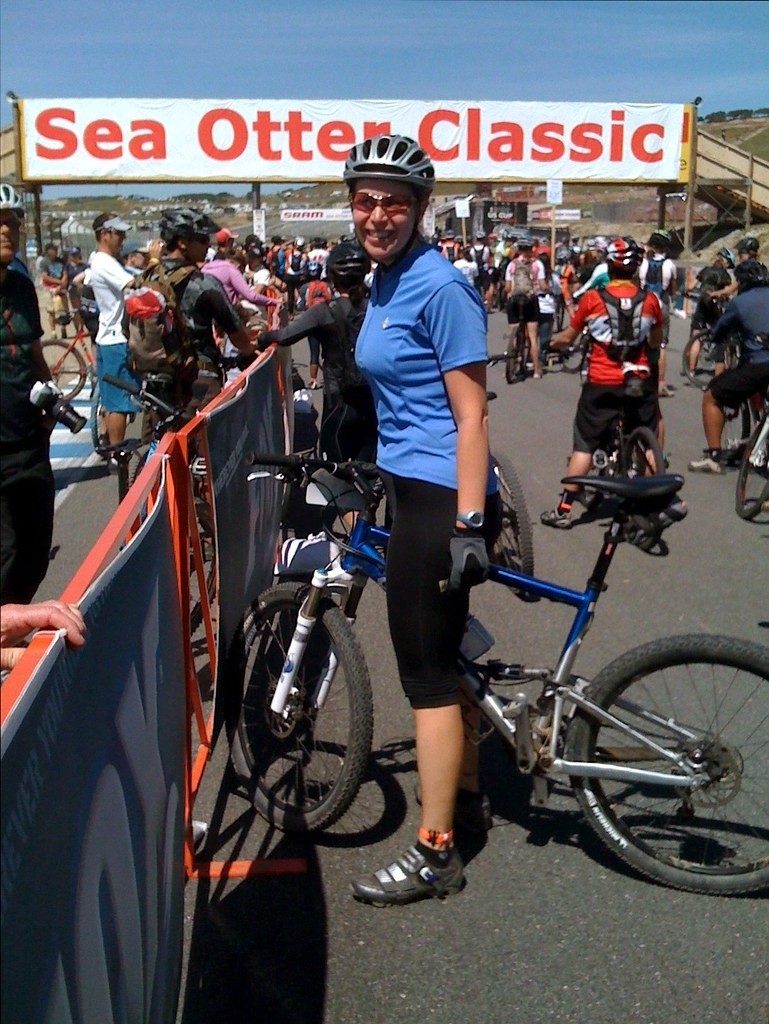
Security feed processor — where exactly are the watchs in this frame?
[456,510,484,529]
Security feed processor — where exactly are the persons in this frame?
[0,182,87,671]
[83,206,254,536]
[344,132,490,907]
[427,230,549,379]
[538,228,685,528]
[34,242,90,340]
[683,236,769,472]
[196,226,379,527]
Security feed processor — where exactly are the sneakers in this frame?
[687,455,723,473]
[540,506,572,528]
[349,845,463,908]
[414,780,490,830]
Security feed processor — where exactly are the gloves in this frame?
[443,526,491,594]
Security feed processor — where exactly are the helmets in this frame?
[343,134,435,190]
[0,184,25,217]
[718,246,736,269]
[606,235,646,273]
[432,224,604,259]
[326,242,370,280]
[734,238,759,251]
[159,206,222,242]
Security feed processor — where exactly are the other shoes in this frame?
[658,386,673,397]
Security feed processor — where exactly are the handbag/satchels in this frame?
[79,297,99,319]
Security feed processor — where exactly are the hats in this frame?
[216,228,239,244]
[95,216,133,232]
[122,238,149,256]
[246,231,350,260]
[69,247,80,254]
[44,242,57,250]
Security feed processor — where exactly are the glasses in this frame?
[348,191,416,214]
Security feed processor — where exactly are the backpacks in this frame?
[512,258,537,296]
[126,263,199,372]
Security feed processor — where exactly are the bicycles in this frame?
[221,451,769,897]
[545,325,666,551]
[254,274,587,592]
[40,313,248,504]
[684,292,768,519]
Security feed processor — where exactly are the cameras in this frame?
[29,381,87,433]
